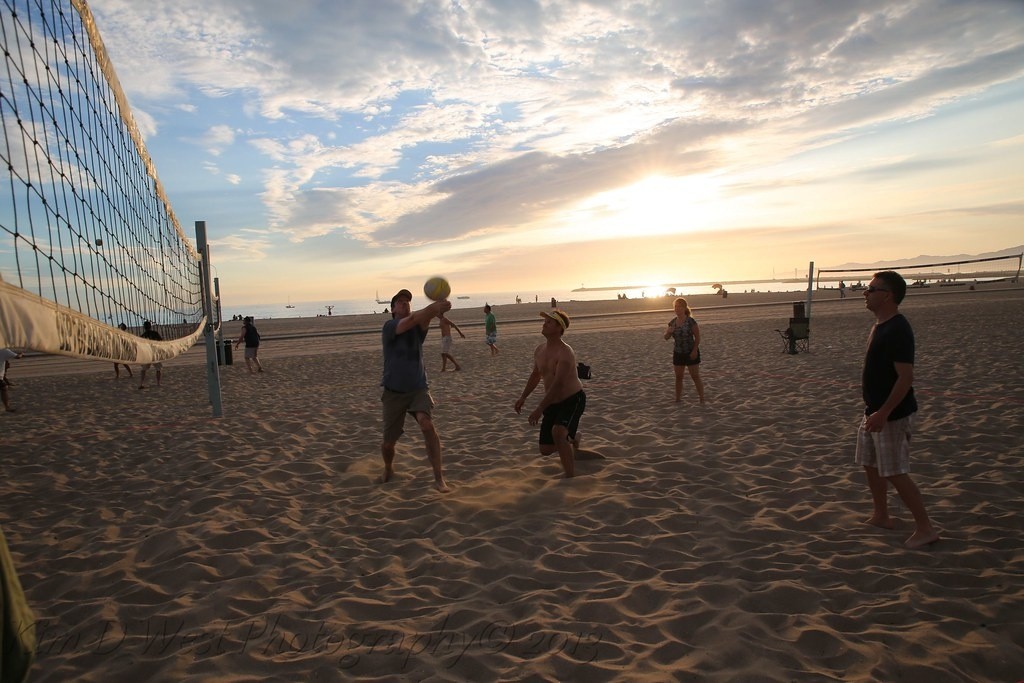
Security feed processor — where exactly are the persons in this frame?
[484,305,500,355]
[840,280,846,298]
[384,308,388,313]
[437,314,465,372]
[233,314,243,321]
[0,348,24,412]
[618,293,627,299]
[852,271,939,548]
[514,310,585,479]
[374,311,376,314]
[138,321,163,389]
[380,289,452,492]
[664,298,705,402]
[535,295,538,303]
[515,294,520,303]
[113,323,133,378]
[235,316,262,372]
[325,305,335,316]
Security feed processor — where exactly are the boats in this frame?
[849,285,868,290]
[906,285,930,288]
[936,281,966,287]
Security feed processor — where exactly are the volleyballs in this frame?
[95,239,102,246]
[423,277,451,301]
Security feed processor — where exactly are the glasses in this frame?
[867,285,884,292]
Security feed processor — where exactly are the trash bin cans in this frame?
[216,340,233,365]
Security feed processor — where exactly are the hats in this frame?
[390,289,412,306]
[244,316,252,322]
[539,311,567,330]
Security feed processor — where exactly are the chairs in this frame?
[773,317,810,354]
